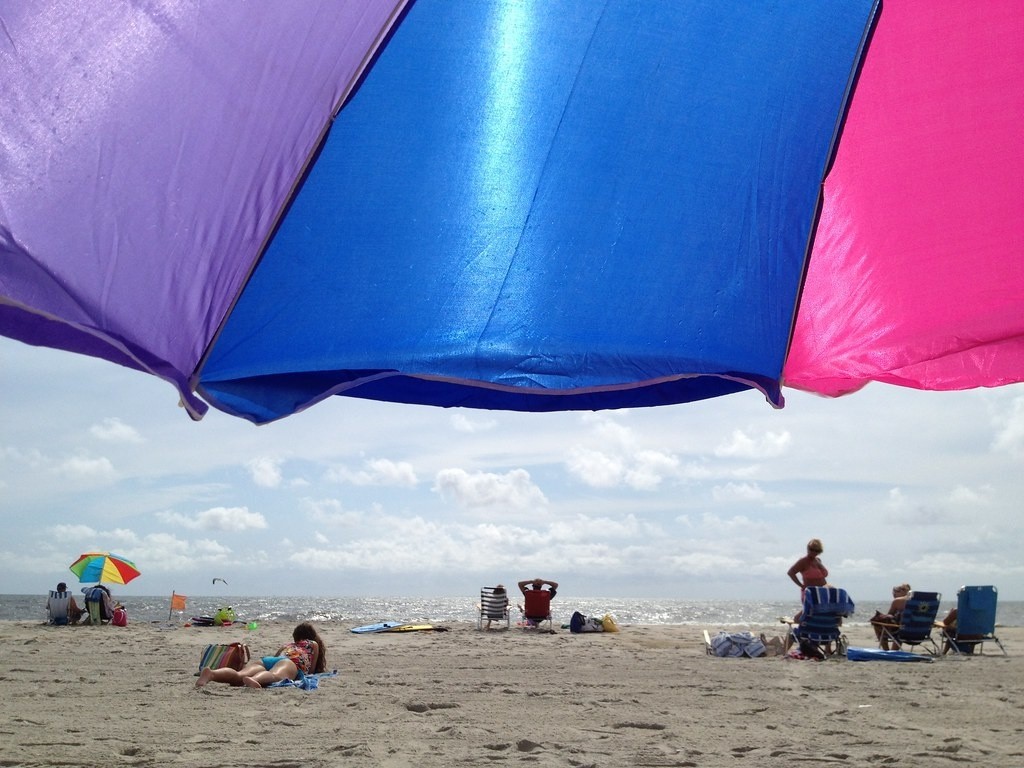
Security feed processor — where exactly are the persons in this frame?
[788,539,835,654]
[517,579,558,625]
[47,583,83,623]
[783,585,832,654]
[943,587,984,654]
[486,585,509,629]
[194,624,326,687]
[874,584,913,650]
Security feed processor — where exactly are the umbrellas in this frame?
[0,0,1023,420]
[70,552,141,586]
[848,647,935,663]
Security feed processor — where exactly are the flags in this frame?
[172,594,187,610]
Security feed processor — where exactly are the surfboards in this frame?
[350,619,448,634]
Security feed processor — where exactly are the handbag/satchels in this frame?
[601,613,620,632]
[113,607,127,627]
[199,642,251,671]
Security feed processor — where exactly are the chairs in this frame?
[83,587,126,626]
[478,586,510,632]
[777,585,855,661]
[868,590,942,659]
[521,589,553,632]
[46,590,76,627]
[934,585,1010,658]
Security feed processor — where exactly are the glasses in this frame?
[809,548,822,553]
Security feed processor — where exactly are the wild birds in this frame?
[213,577,228,585]
